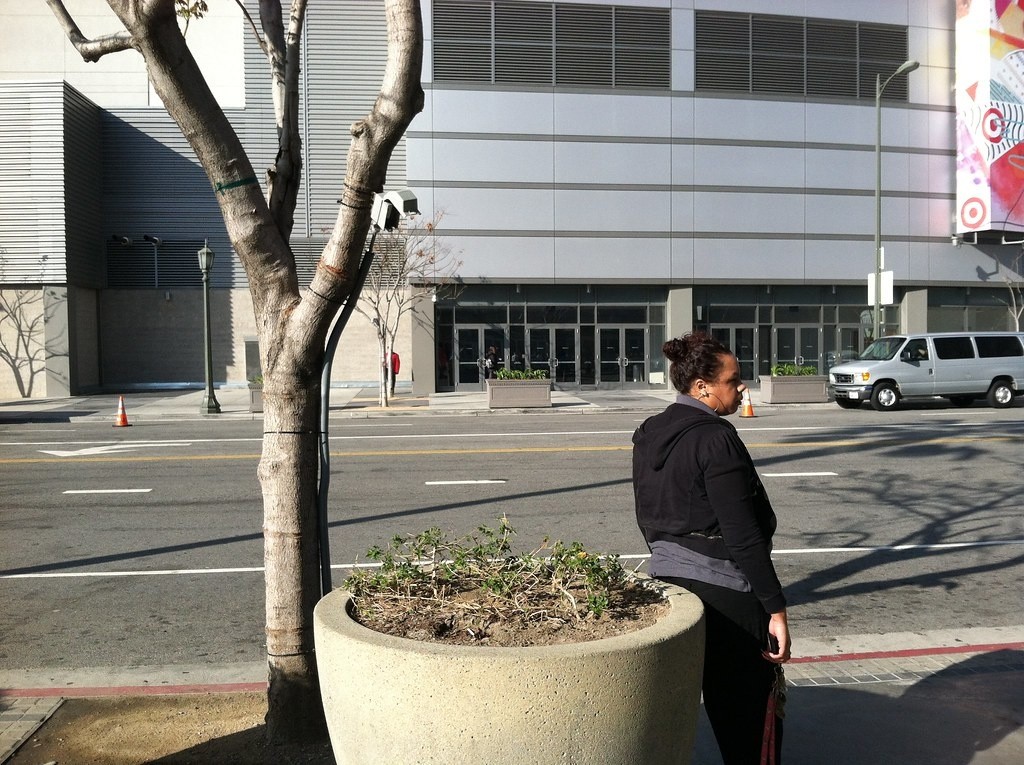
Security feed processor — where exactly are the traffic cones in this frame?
[741,389,757,418]
[114,397,133,428]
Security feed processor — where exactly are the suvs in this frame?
[825,339,874,369]
[826,330,1024,410]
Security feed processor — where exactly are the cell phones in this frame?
[766,633,780,655]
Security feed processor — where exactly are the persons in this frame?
[383,346,400,394]
[911,345,926,359]
[631,332,791,765]
[485,346,497,379]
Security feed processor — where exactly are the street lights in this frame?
[872,60,920,358]
[318,189,420,595]
[198,239,222,413]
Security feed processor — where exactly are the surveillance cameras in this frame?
[952,237,959,246]
